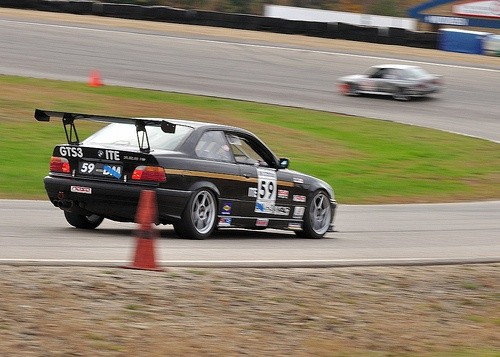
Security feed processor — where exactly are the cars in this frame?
[33,109,337,240]
[337,65,443,100]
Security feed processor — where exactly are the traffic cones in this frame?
[86,68,104,87]
[125,234,162,273]
